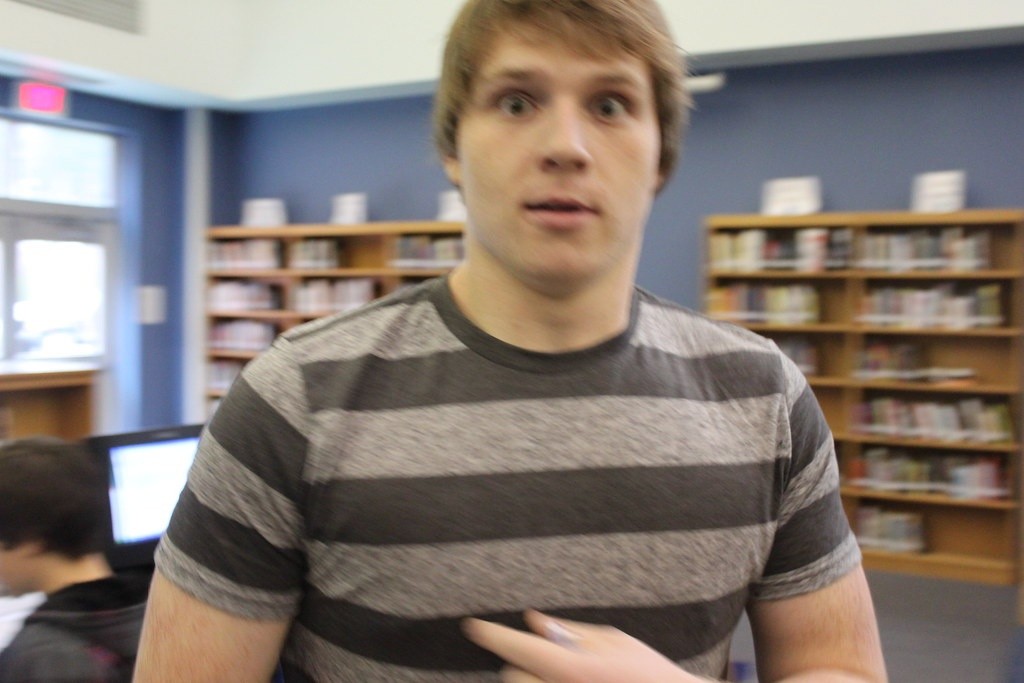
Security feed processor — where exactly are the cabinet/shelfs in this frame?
[204,220,465,424]
[699,211,1024,583]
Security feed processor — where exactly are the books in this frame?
[711,168,1015,554]
[210,189,464,410]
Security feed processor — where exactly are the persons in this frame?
[134,0,892,682]
[1,435,148,683]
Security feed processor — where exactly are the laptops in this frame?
[84,423,206,572]
